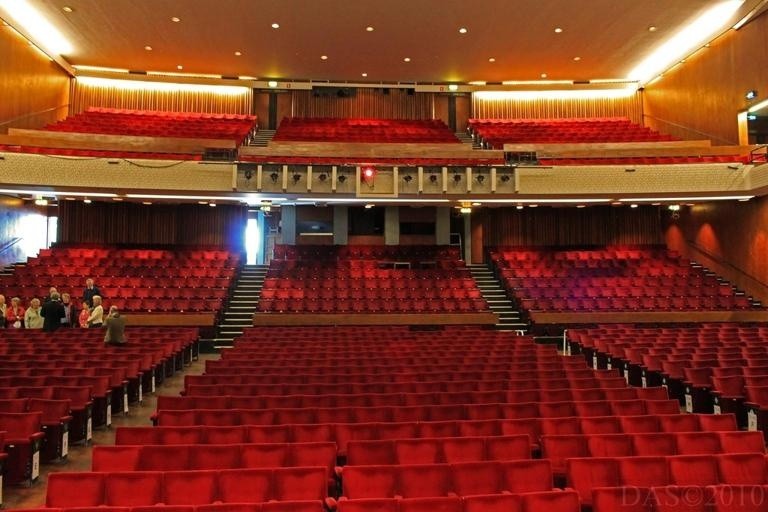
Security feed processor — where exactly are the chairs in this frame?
[0,106,768,165]
[1,241,768,511]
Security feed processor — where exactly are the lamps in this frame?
[244,171,510,184]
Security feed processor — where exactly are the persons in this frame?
[0,278,104,330]
[101,305,128,347]
